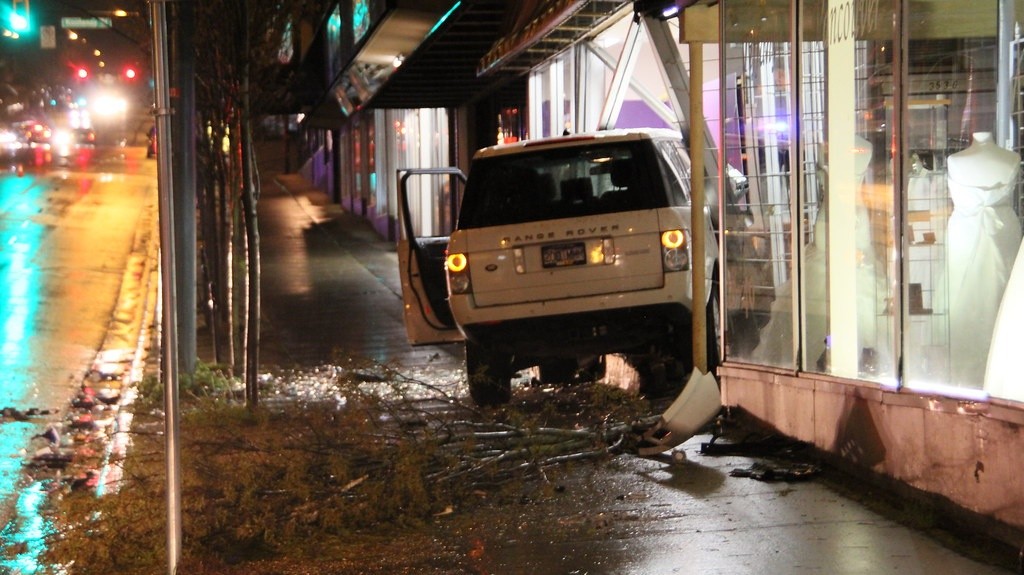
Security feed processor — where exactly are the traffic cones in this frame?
[18,164,23,177]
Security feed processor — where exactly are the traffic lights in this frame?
[12,0,28,31]
[78,69,88,80]
[126,69,135,79]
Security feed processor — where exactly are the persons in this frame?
[927,130,1022,388]
[813,132,878,368]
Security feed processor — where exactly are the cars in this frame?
[723,134,811,314]
[396,127,726,406]
